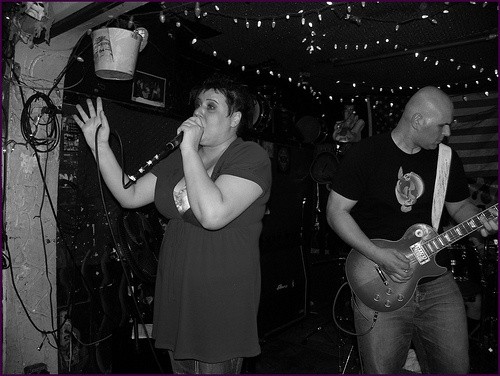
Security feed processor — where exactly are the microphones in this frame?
[149,130,184,167]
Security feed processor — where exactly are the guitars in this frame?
[345,202,500,313]
[309,151,336,185]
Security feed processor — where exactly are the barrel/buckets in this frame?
[92,16,143,81]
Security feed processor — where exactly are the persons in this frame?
[326,86,499,374]
[73,76,271,375]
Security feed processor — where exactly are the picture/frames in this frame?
[131,70,167,108]
